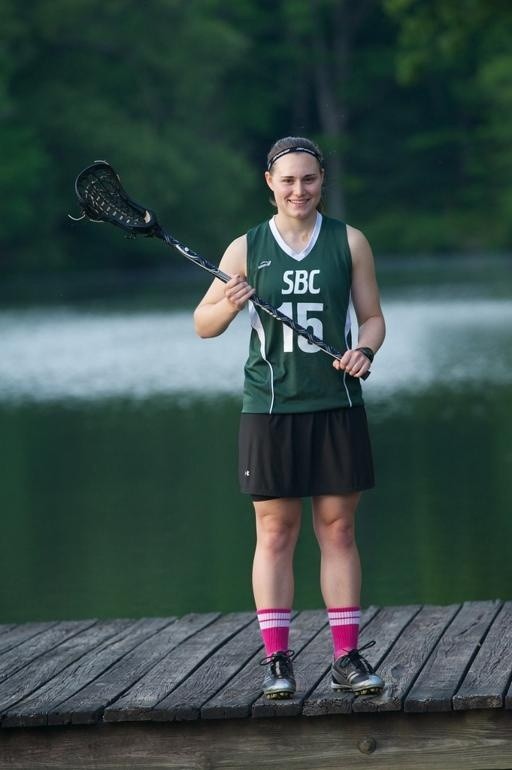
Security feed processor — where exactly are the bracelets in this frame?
[353,345,375,362]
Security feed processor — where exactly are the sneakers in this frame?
[263,650,295,700]
[331,650,385,694]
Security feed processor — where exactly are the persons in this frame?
[191,139,394,698]
